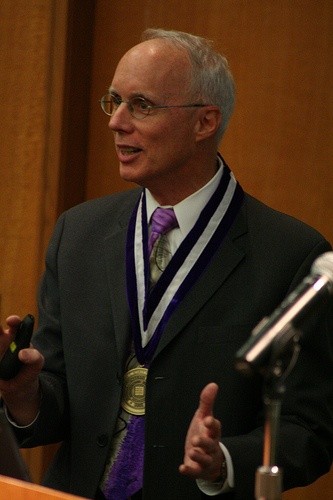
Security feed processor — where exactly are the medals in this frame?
[121,367,148,416]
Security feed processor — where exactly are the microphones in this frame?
[236,251,333,376]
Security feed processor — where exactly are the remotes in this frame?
[0,314,35,380]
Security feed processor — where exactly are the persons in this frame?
[0,28,333,500]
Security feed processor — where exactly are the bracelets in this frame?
[211,462,226,483]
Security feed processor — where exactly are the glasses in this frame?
[96,93,207,120]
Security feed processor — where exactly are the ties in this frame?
[98,206,181,500]
